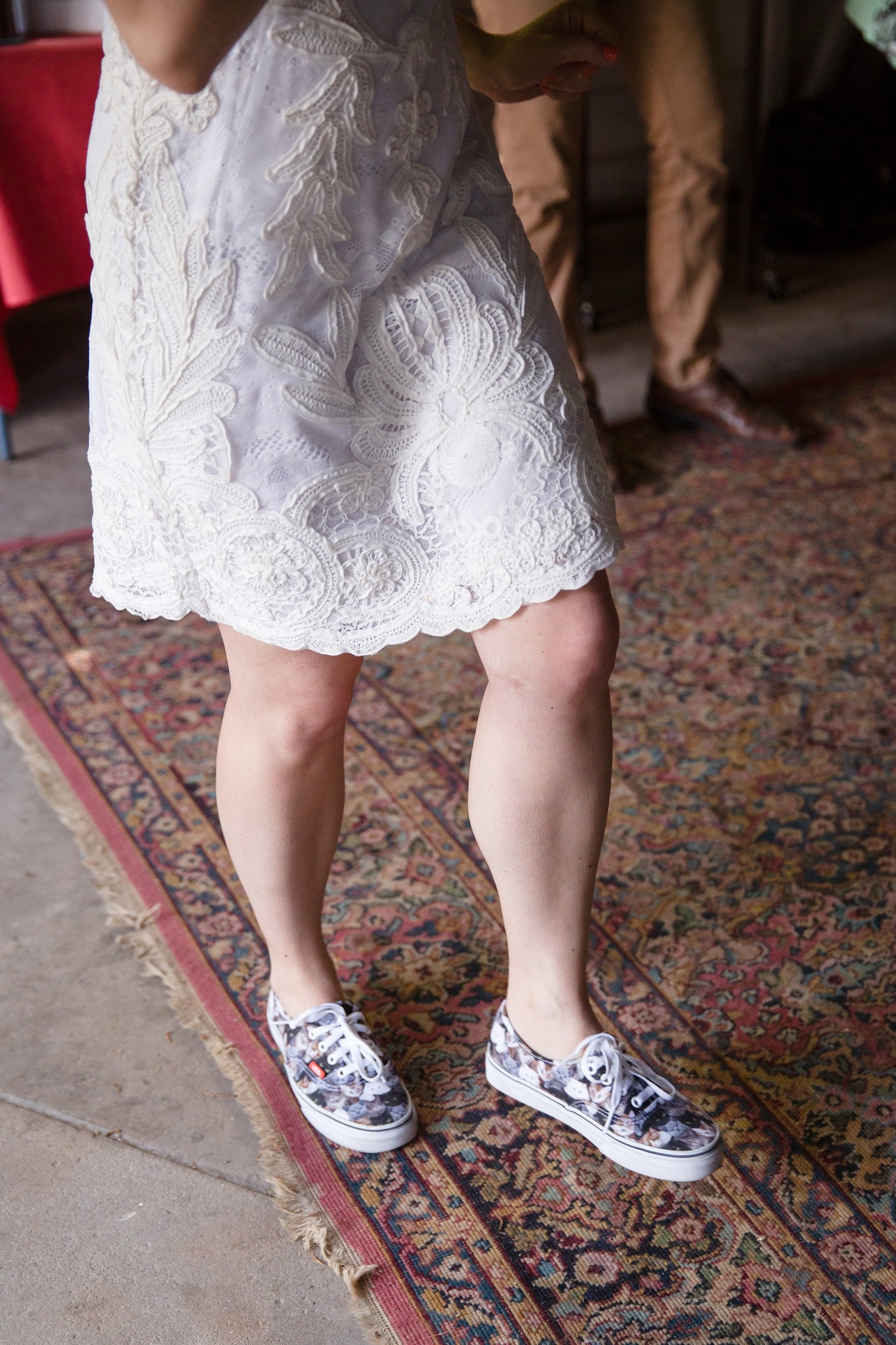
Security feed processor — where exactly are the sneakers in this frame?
[265,988,420,1154]
[484,999,726,1183]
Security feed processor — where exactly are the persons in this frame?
[473,0,817,492]
[80,0,732,1187]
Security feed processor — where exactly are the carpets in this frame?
[3,343,896,1342]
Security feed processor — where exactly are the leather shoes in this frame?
[645,362,811,450]
[584,394,625,491]
